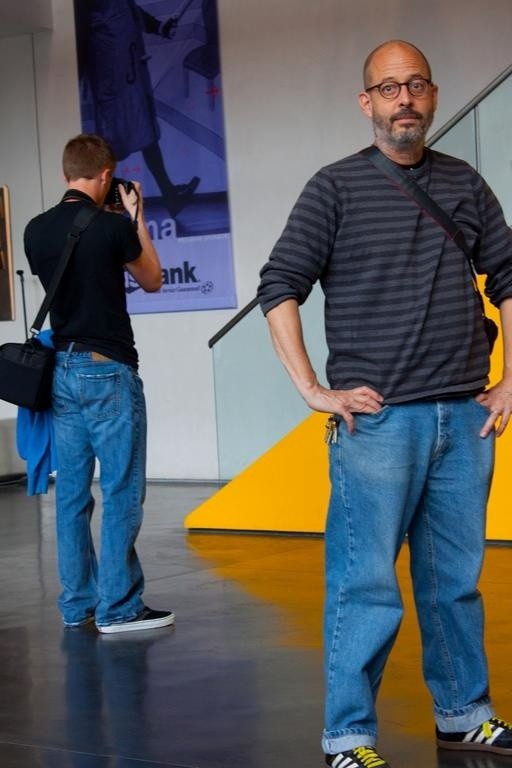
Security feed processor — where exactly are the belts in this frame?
[56,342,94,352]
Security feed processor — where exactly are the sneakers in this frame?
[324,745,392,768]
[99,608,178,634]
[435,716,512,756]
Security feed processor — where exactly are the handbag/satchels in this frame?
[0,338,55,412]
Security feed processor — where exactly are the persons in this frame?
[259,36,512,767]
[74,0,201,220]
[22,132,176,634]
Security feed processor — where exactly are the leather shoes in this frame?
[165,176,200,219]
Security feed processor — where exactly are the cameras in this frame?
[103,177,132,205]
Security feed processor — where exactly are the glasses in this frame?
[365,78,433,99]
